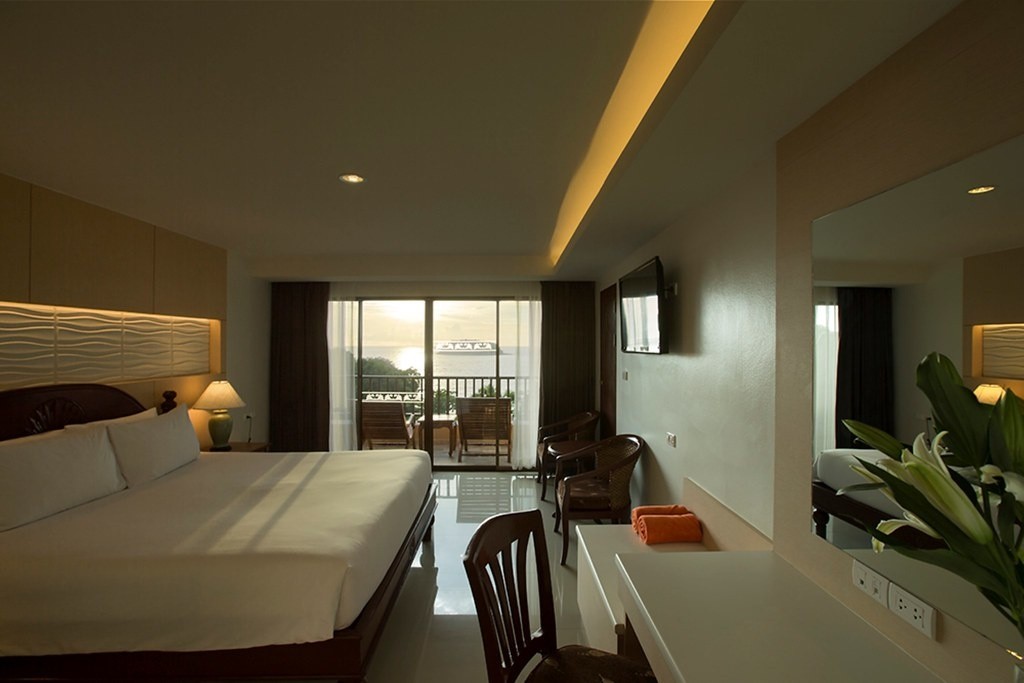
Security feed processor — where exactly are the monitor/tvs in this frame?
[619,255,669,355]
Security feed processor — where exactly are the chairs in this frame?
[461,509,656,683]
[455,398,513,463]
[554,434,643,565]
[355,400,416,449]
[536,410,600,500]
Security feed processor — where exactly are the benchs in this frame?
[574,523,708,653]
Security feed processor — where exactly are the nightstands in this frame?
[201,442,273,452]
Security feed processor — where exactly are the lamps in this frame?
[972,383,1007,405]
[193,380,246,452]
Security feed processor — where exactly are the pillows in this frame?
[107,403,200,488]
[0,424,128,532]
[64,406,158,428]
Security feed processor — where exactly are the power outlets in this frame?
[667,282,677,297]
[926,414,931,423]
[888,581,937,640]
[244,412,254,421]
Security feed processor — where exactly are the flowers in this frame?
[835,352,1024,638]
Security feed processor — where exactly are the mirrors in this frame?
[811,134,1024,660]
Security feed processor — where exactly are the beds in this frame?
[812,449,1024,549]
[0,382,438,683]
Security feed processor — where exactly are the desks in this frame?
[548,440,597,456]
[613,549,949,682]
[417,414,458,457]
